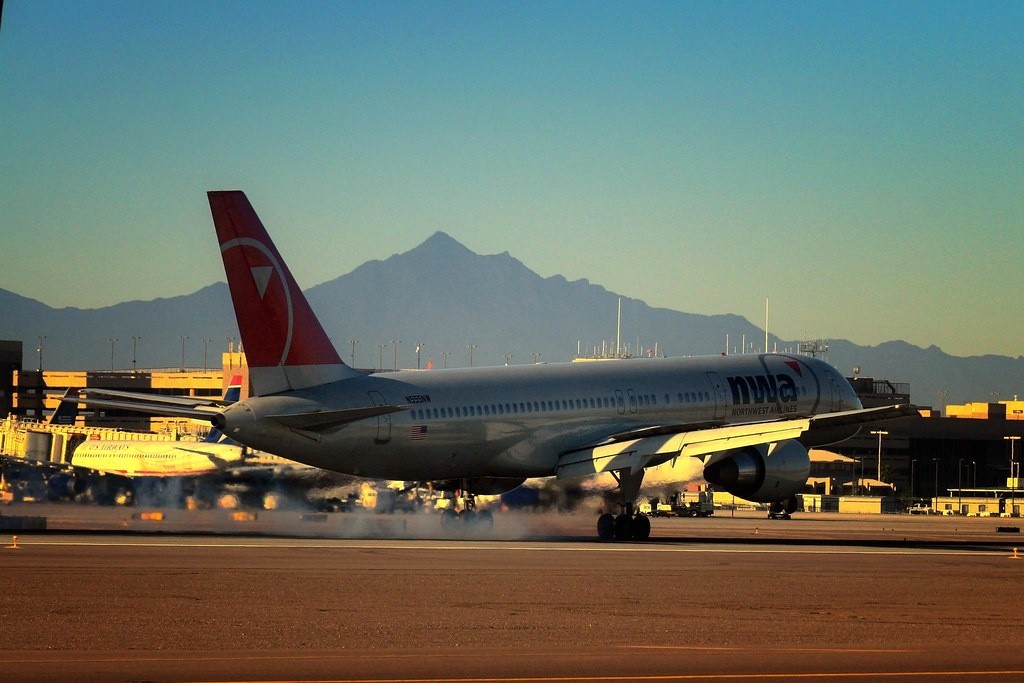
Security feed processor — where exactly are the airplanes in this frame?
[49,190,865,541]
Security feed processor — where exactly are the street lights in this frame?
[178,336,189,372]
[870,429,889,482]
[911,459,917,509]
[201,339,212,374]
[38,335,47,372]
[224,336,235,353]
[972,461,977,498]
[1003,436,1023,517]
[328,333,542,375]
[959,459,964,515]
[109,338,118,375]
[131,336,141,371]
[933,458,942,515]
